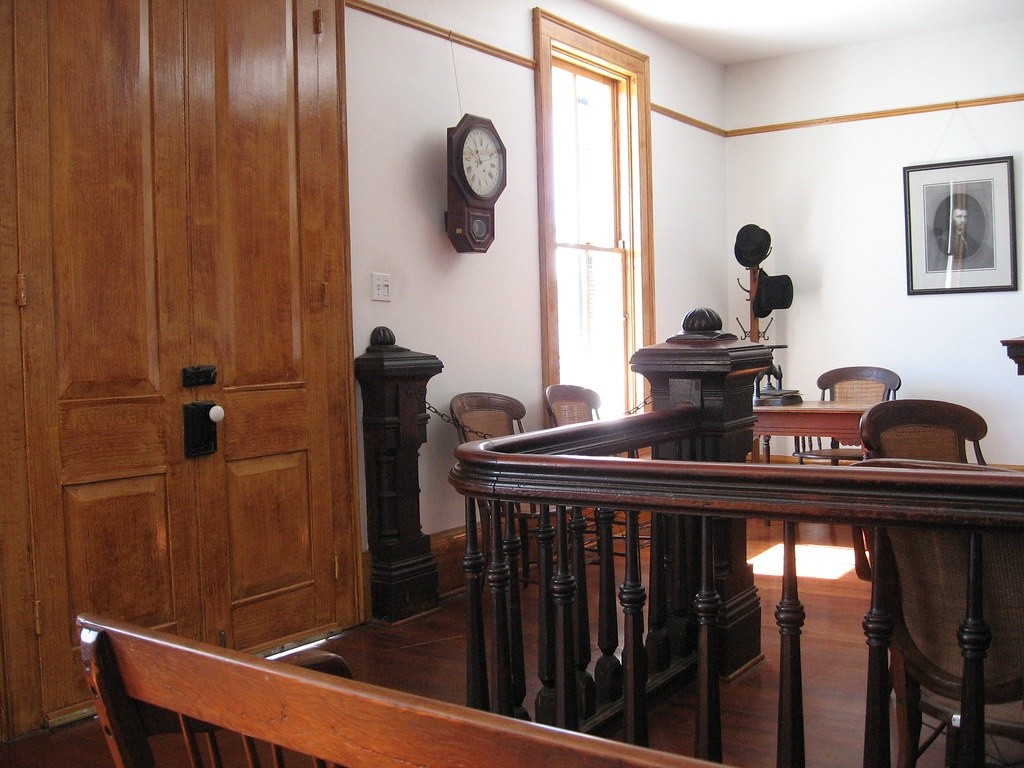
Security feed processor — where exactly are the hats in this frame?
[734,224,771,267]
[753,270,794,318]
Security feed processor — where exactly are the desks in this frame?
[754,401,877,527]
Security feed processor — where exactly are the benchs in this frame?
[76,613,724,768]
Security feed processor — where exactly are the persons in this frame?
[935,205,979,260]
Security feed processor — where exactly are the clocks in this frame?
[444,113,507,253]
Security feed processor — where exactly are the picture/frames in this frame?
[903,156,1018,295]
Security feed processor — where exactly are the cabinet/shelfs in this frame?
[0,0,368,740]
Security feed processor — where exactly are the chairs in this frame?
[859,399,989,466]
[544,384,651,558]
[450,391,576,588]
[849,459,1024,768]
[792,367,902,466]
